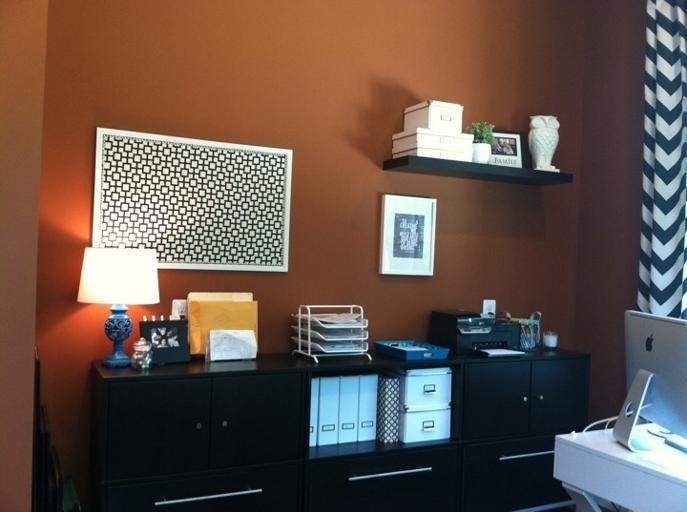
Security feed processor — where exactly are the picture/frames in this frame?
[91,126,294,276]
[491,128,526,170]
[378,193,438,278]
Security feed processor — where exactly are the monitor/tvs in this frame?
[607,308,687,458]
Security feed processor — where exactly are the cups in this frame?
[542,331,559,348]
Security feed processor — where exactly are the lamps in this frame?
[76,247,160,370]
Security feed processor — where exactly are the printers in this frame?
[429,318,525,359]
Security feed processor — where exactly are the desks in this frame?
[553,420,687,512]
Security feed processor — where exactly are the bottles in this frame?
[131,338,153,368]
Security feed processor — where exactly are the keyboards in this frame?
[661,433,687,453]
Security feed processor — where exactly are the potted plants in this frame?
[462,119,495,167]
